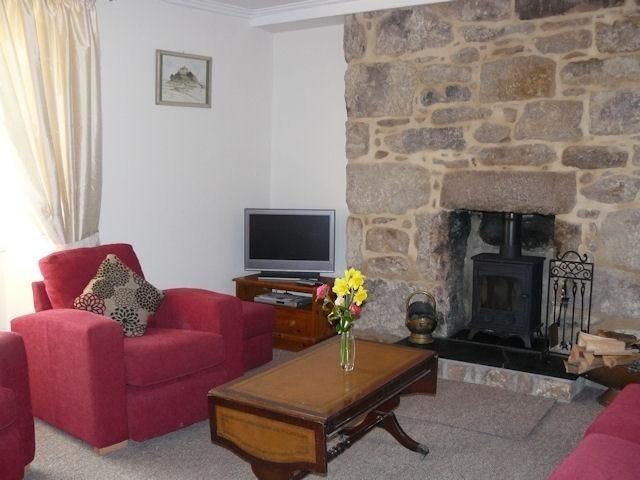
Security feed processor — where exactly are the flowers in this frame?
[315,267,369,365]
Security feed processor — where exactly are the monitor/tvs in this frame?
[242,208,336,280]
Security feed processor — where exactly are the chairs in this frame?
[548,381,639,480]
[11,243,246,456]
[0,329,37,480]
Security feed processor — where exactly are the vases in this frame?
[340,330,356,372]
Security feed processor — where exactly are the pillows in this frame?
[75,253,167,337]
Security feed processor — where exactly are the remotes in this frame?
[296,279,316,286]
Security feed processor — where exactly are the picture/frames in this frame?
[154,49,213,107]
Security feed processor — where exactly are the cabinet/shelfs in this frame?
[230,273,335,353]
